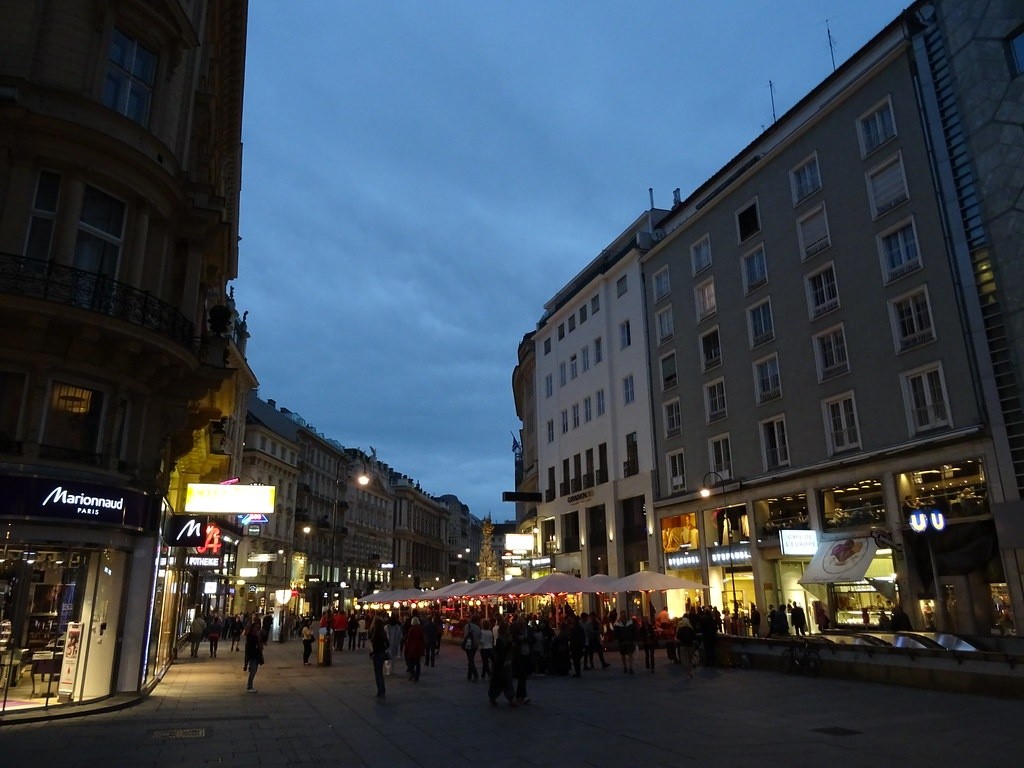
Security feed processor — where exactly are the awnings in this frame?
[797,535,882,583]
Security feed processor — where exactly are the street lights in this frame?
[324,453,370,667]
[700,471,739,637]
[280,514,311,643]
[263,543,284,614]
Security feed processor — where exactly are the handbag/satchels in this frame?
[461,624,473,651]
[185,634,191,641]
[310,635,315,643]
[208,632,219,641]
[384,648,392,660]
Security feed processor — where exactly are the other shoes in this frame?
[415,677,418,681]
[248,688,257,693]
[573,674,581,677]
[304,663,311,666]
[624,667,627,672]
[583,666,591,670]
[489,698,498,706]
[629,670,633,674]
[603,664,610,668]
[408,672,415,681]
[523,698,530,705]
[509,701,519,708]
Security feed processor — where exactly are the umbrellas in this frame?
[361,571,711,619]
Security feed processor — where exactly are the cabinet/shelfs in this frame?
[0,615,62,699]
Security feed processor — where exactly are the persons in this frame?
[862,605,914,631]
[189,599,832,709]
